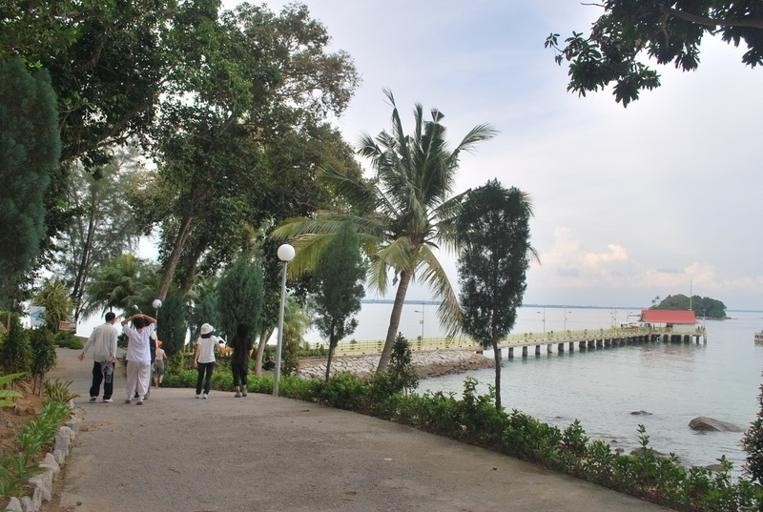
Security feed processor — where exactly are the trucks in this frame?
[30,304,76,336]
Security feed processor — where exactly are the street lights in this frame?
[150,298,162,321]
[536,311,546,336]
[270,243,296,398]
[414,309,425,342]
[564,311,571,332]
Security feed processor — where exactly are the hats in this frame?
[158,340,163,347]
[200,322,213,335]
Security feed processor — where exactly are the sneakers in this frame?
[194,391,248,399]
[88,394,151,405]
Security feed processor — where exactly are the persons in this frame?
[77,312,168,405]
[194,323,254,399]
